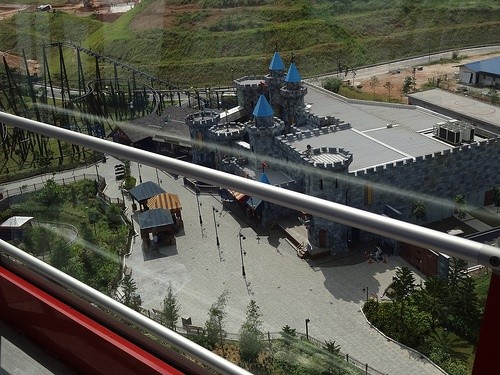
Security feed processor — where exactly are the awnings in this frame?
[128,171,255,235]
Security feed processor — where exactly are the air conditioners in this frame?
[436,124,462,144]
[458,126,475,142]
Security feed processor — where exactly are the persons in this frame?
[375,245,383,258]
[305,144,314,158]
